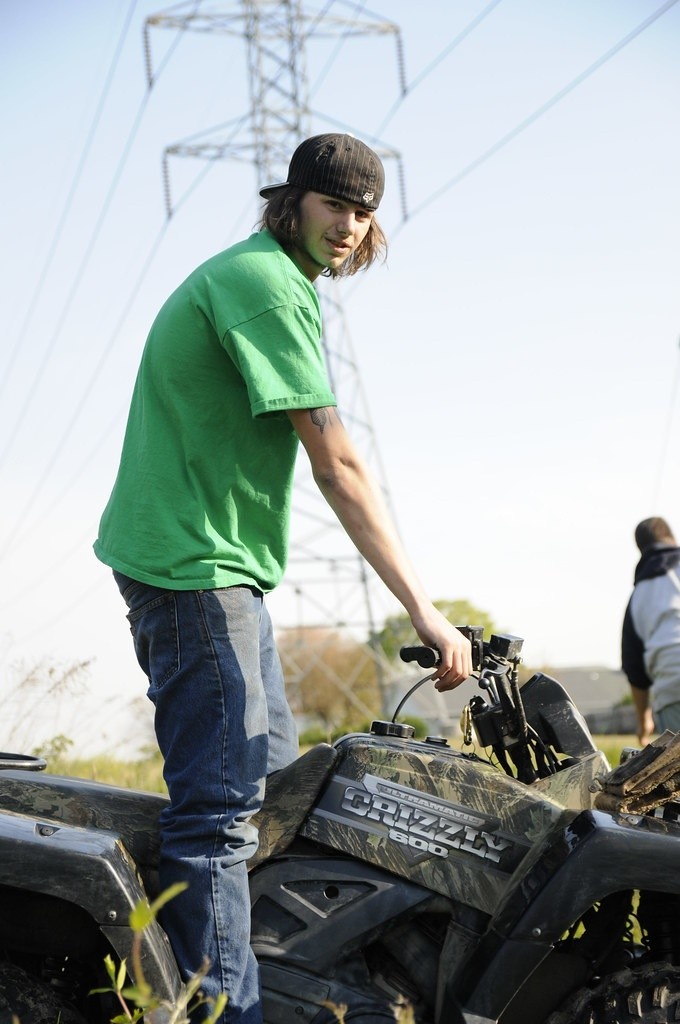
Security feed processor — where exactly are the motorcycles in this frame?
[0,626,680,1024]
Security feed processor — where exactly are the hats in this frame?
[259,133,385,211]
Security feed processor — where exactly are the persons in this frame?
[94,133,475,1024]
[620,516,680,746]
